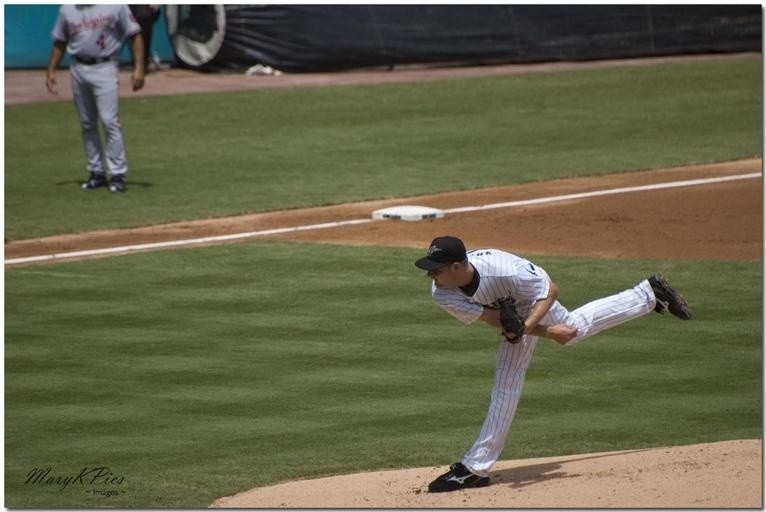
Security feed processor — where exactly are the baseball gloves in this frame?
[499,304,525,343]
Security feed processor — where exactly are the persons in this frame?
[126,5,161,75]
[42,4,147,194]
[412,233,693,494]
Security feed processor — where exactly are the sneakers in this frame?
[648,272,692,321]
[79,175,128,193]
[428,462,490,492]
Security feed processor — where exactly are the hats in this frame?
[414,236,467,271]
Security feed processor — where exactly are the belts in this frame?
[72,55,110,65]
